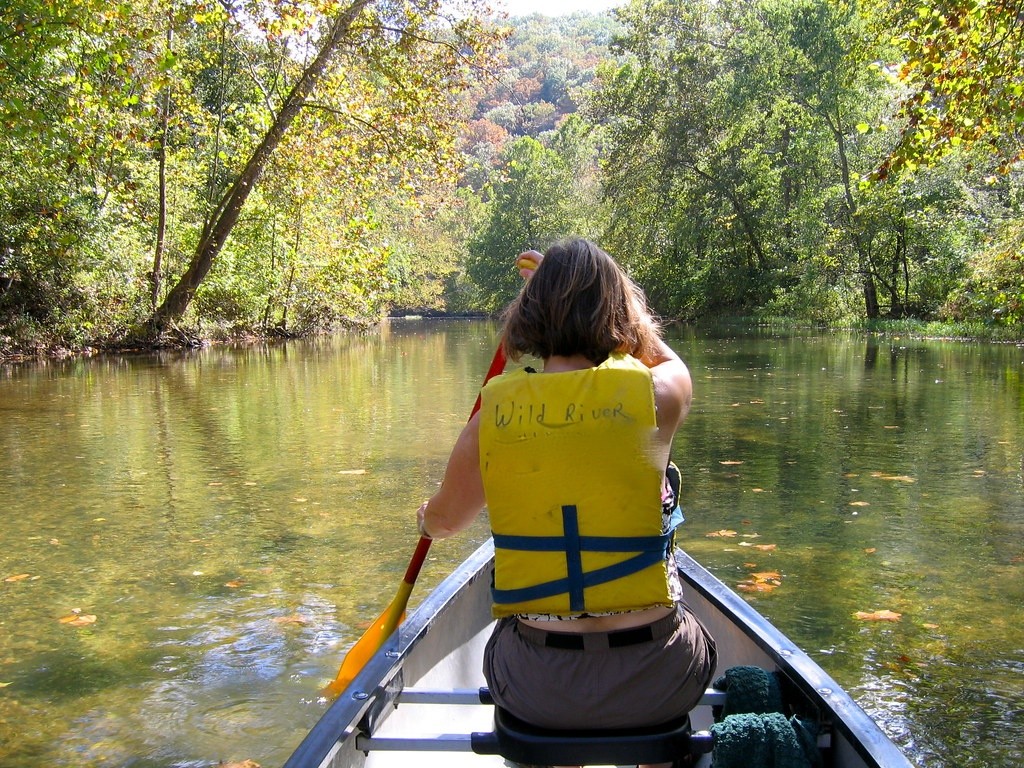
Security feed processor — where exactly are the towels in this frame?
[709,712,824,768]
[713,664,785,721]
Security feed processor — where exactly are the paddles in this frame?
[332,258,545,685]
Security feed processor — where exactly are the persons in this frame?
[416,237,714,730]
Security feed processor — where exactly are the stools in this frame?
[471,678,698,768]
